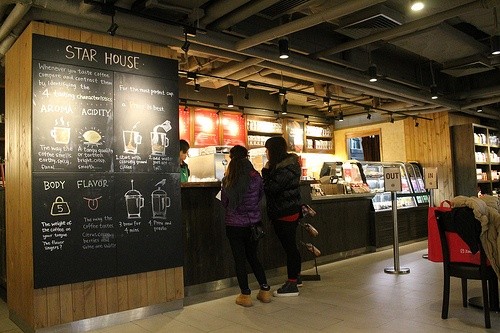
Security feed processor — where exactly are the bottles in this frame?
[302,205,316,218]
[299,240,321,257]
[298,221,319,238]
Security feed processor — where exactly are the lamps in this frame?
[239,107,245,118]
[279,65,288,115]
[364,106,371,119]
[187,73,200,92]
[180,99,188,111]
[477,106,482,112]
[239,82,250,100]
[366,64,378,82]
[182,28,196,52]
[274,111,279,121]
[226,85,233,107]
[214,103,220,115]
[491,7,500,54]
[388,111,394,123]
[412,117,419,127]
[279,36,288,59]
[304,115,309,125]
[323,98,332,113]
[339,105,344,119]
[101,6,118,36]
[430,61,438,99]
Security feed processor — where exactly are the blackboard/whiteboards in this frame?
[30,32,186,290]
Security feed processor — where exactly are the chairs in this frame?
[434,209,500,328]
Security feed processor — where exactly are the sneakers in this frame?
[297,276,303,287]
[274,280,300,296]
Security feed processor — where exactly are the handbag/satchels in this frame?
[427,200,493,267]
[250,224,271,241]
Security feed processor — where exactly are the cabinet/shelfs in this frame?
[450,123,500,199]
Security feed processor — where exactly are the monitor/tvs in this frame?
[321,162,344,184]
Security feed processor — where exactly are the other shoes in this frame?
[237,293,254,307]
[257,286,272,303]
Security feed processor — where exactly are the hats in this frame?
[180,139,190,153]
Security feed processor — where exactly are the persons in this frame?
[221,144,271,306]
[180,139,191,182]
[261,136,304,297]
